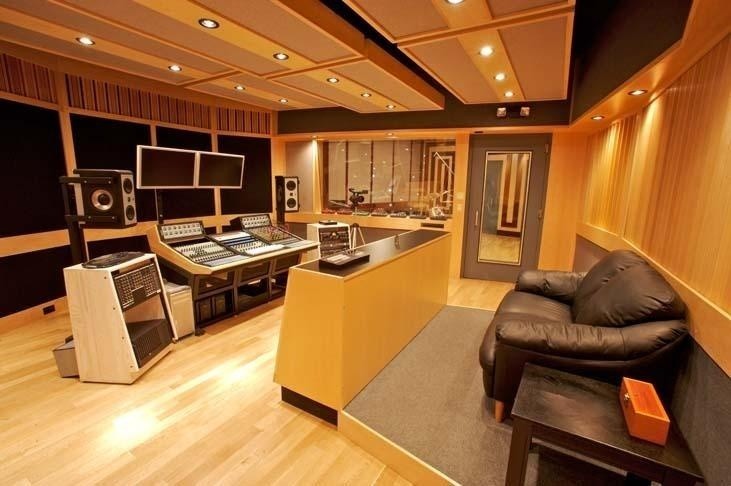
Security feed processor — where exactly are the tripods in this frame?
[350,206,365,247]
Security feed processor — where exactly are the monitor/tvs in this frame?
[136,145,197,188]
[196,151,245,190]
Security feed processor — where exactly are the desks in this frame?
[504,358,706,486]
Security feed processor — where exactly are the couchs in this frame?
[479,249,701,421]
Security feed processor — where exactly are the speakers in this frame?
[276,176,300,211]
[73,168,137,228]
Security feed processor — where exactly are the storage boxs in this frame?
[619,375,669,447]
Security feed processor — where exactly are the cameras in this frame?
[350,195,364,203]
